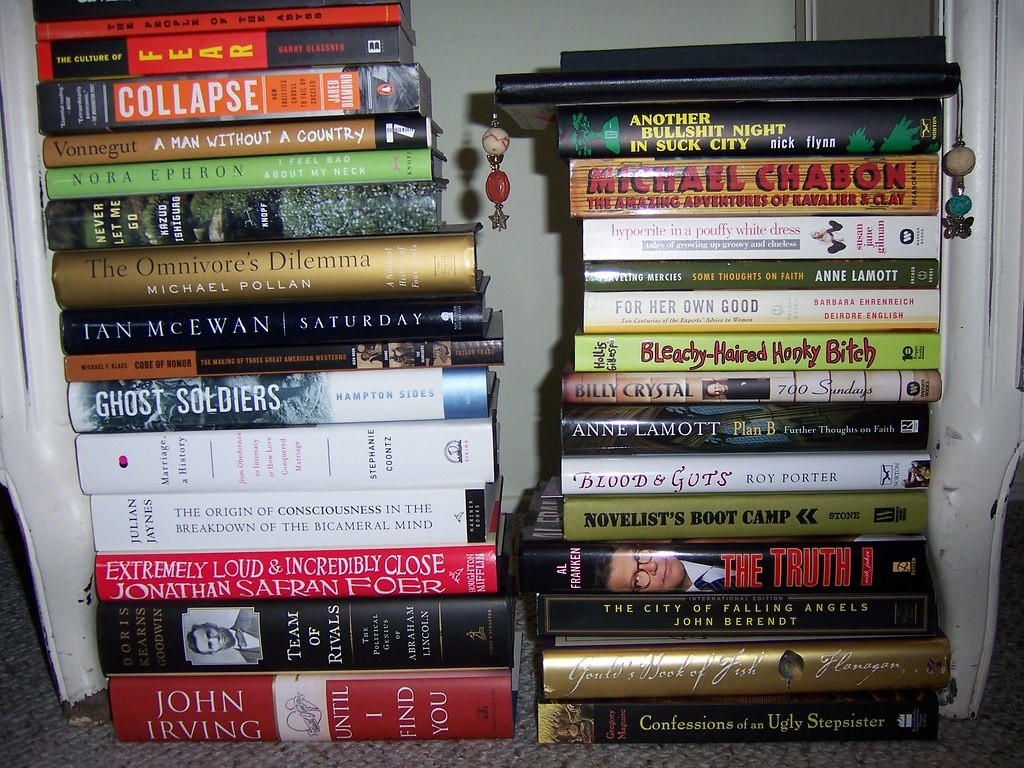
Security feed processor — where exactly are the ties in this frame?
[694,576,738,591]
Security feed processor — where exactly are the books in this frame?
[33,0,952,742]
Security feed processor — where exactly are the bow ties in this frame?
[235,629,247,650]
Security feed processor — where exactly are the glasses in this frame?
[631,548,653,593]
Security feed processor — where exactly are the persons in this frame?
[361,352,383,368]
[705,378,770,399]
[186,606,262,663]
[395,351,416,365]
[433,345,451,365]
[366,343,382,355]
[581,542,774,595]
